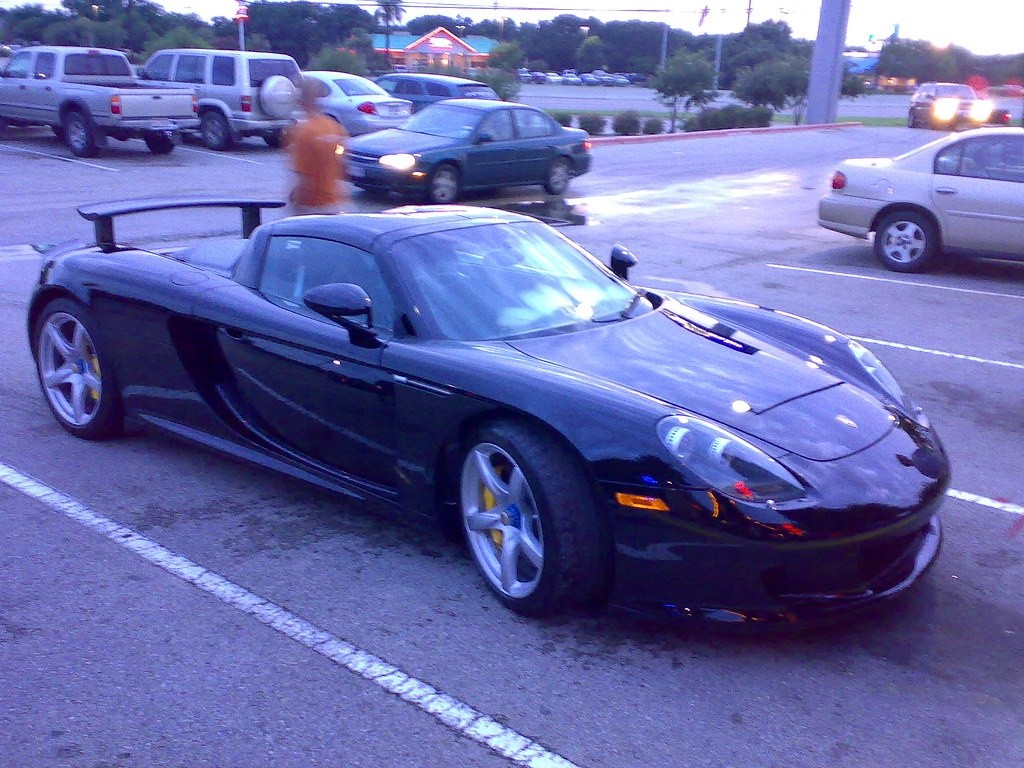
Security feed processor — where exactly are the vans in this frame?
[394,65,409,73]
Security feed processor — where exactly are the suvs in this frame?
[0,43,26,71]
[134,49,308,151]
[978,84,1024,123]
[908,81,984,129]
[518,69,533,83]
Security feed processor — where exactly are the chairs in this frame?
[937,155,990,179]
[303,245,393,330]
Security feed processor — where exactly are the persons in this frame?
[288,79,346,216]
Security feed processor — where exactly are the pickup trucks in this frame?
[0,45,202,157]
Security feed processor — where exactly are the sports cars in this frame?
[27,198,953,628]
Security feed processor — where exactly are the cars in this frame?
[297,73,412,136]
[530,72,546,82]
[561,74,581,85]
[816,126,1024,274]
[369,74,503,116]
[544,73,562,84]
[340,98,592,203]
[565,69,644,85]
